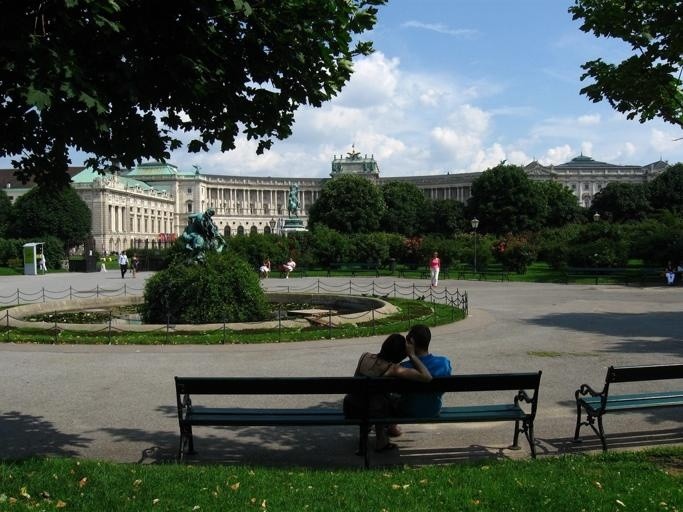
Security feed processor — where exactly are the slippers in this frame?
[375,442,396,453]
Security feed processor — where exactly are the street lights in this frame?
[591,209,600,223]
[268,217,277,233]
[469,214,480,273]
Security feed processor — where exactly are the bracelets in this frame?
[410,354,415,359]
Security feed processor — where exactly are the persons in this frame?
[182,207,226,261]
[282,256,296,279]
[39,250,48,272]
[259,256,271,279]
[382,323,451,437]
[130,252,140,278]
[344,332,432,456]
[664,260,676,285]
[428,251,441,287]
[288,184,299,205]
[118,250,129,279]
